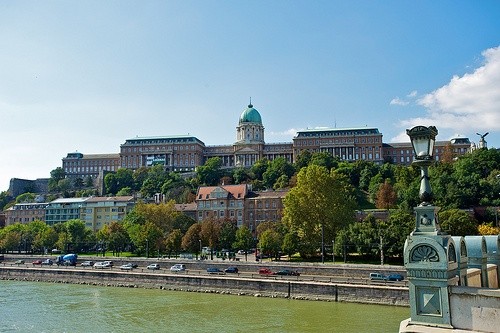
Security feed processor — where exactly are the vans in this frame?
[369,272,404,282]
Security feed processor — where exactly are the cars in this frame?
[207,267,224,275]
[52,249,59,254]
[276,269,296,275]
[32,259,54,265]
[238,250,244,255]
[119,263,138,270]
[170,264,186,272]
[92,262,106,269]
[260,269,275,276]
[147,264,160,270]
[104,261,114,268]
[224,266,239,274]
[15,259,25,264]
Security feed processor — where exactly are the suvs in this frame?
[80,261,94,267]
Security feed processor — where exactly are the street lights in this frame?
[404,125,442,237]
